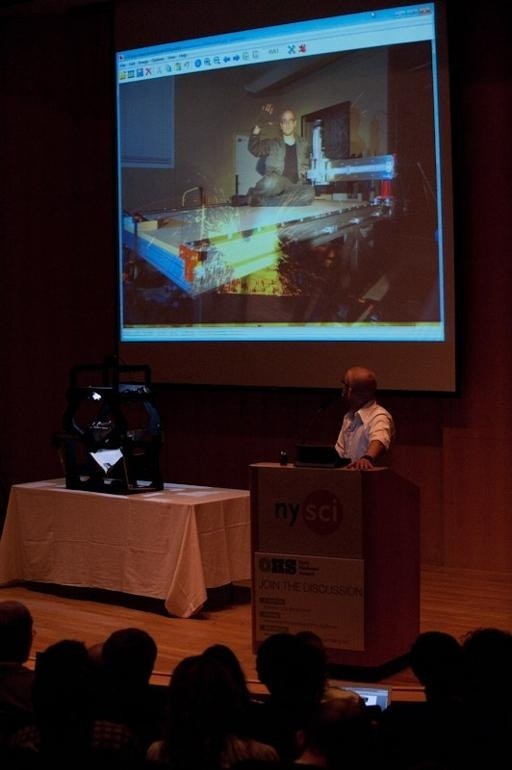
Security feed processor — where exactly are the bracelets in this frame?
[360,455,374,462]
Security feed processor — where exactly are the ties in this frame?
[343,416,363,459]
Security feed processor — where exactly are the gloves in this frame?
[254,109,272,128]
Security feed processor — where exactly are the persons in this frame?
[229,102,319,209]
[334,365,397,473]
[0,600,511,769]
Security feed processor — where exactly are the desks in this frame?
[0,475,250,610]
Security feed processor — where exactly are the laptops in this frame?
[338,682,392,711]
[294,443,352,467]
